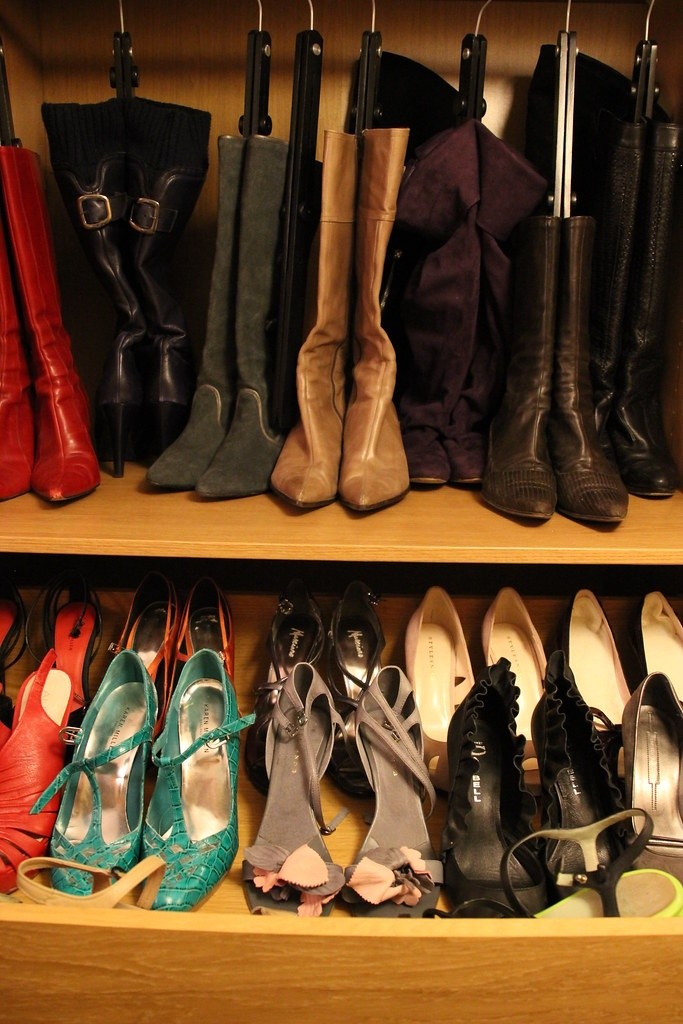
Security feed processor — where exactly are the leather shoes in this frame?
[441,657,546,918]
[620,674,683,885]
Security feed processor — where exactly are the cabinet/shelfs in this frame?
[0,463,683,1024]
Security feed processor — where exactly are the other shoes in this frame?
[556,588,634,838]
[627,588,683,717]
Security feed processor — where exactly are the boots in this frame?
[42,98,146,478]
[147,135,248,498]
[128,97,212,456]
[271,127,359,511]
[0,146,101,501]
[550,215,633,522]
[396,118,480,484]
[347,129,411,512]
[0,223,35,504]
[442,120,548,482]
[482,216,561,523]
[608,122,683,496]
[195,136,287,498]
[589,122,647,468]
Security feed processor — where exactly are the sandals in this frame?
[404,585,477,791]
[243,661,346,916]
[344,666,443,918]
[0,574,44,729]
[0,648,76,893]
[245,577,332,791]
[481,587,548,797]
[97,571,181,739]
[142,648,256,911]
[28,650,159,895]
[397,809,683,918]
[26,569,102,767]
[162,574,242,739]
[532,650,624,896]
[326,580,386,797]
[9,855,166,910]
